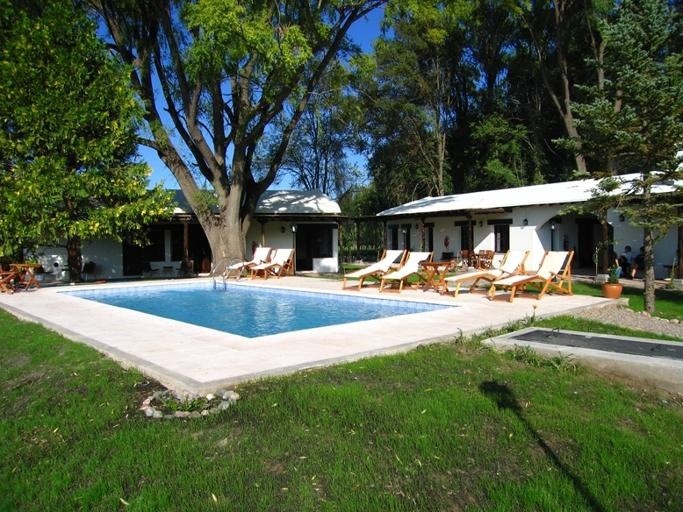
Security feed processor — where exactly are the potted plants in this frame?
[601,263,623,299]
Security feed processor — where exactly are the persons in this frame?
[614,245,631,278]
[51,263,62,280]
[631,246,647,279]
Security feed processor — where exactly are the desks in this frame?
[420,260,452,293]
[0,270,16,295]
[10,263,41,292]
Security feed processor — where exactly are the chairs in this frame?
[488,250,575,302]
[250,249,295,280]
[344,249,407,290]
[379,251,434,293]
[440,249,527,296]
[468,249,545,299]
[224,247,271,280]
